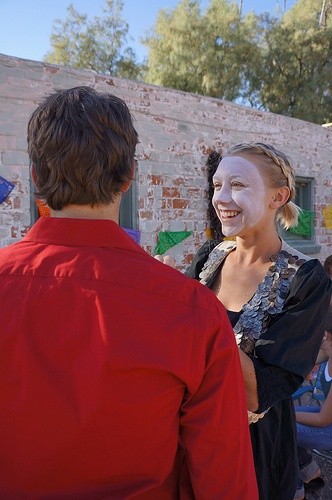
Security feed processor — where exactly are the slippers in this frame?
[304,477,325,495]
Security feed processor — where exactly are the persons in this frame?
[152,142,332,499]
[1,86,259,499]
[293,321,331,500]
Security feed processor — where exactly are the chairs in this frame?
[292,377,321,407]
